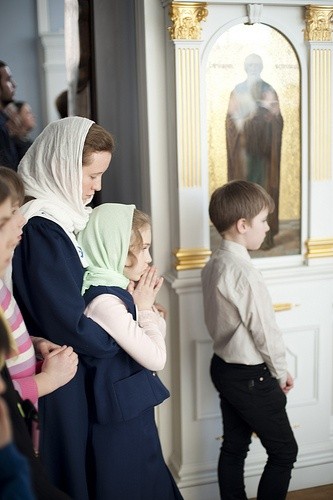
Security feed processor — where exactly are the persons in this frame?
[0,60,36,171]
[225,54,284,250]
[201,179,298,500]
[9,115,122,500]
[80,201,187,499]
[0,166,79,500]
